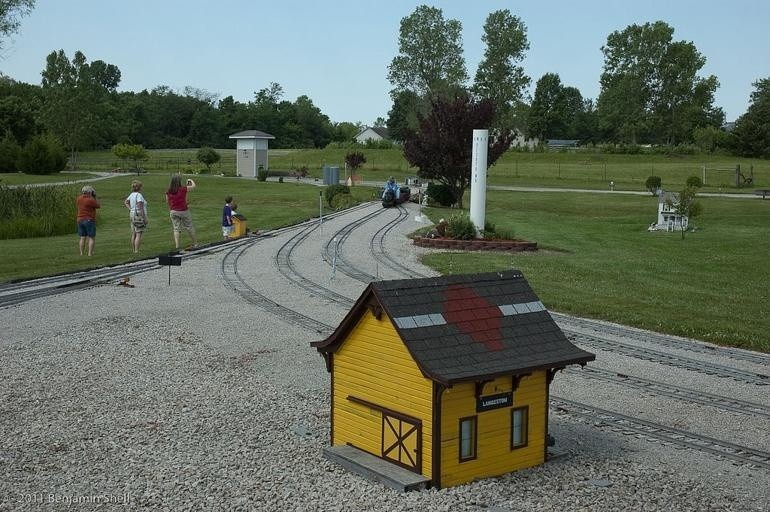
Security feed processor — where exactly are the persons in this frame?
[221,195,233,240]
[230,204,238,216]
[124,179,148,252]
[165,173,200,250]
[73,184,99,258]
[382,176,398,200]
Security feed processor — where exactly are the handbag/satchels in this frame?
[132,213,147,227]
